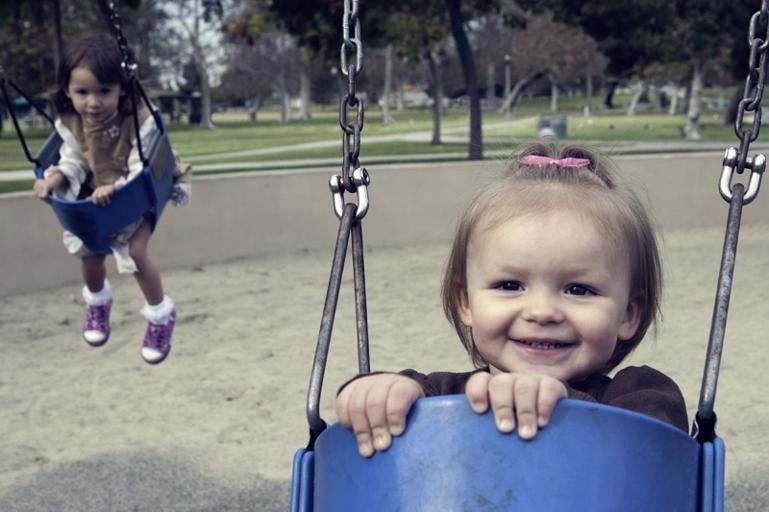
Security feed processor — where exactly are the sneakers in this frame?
[141,307,177,364]
[82,297,114,347]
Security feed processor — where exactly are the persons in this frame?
[33,33,177,363]
[334,142,688,458]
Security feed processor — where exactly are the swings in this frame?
[290,2,769,511]
[0,2,179,254]
